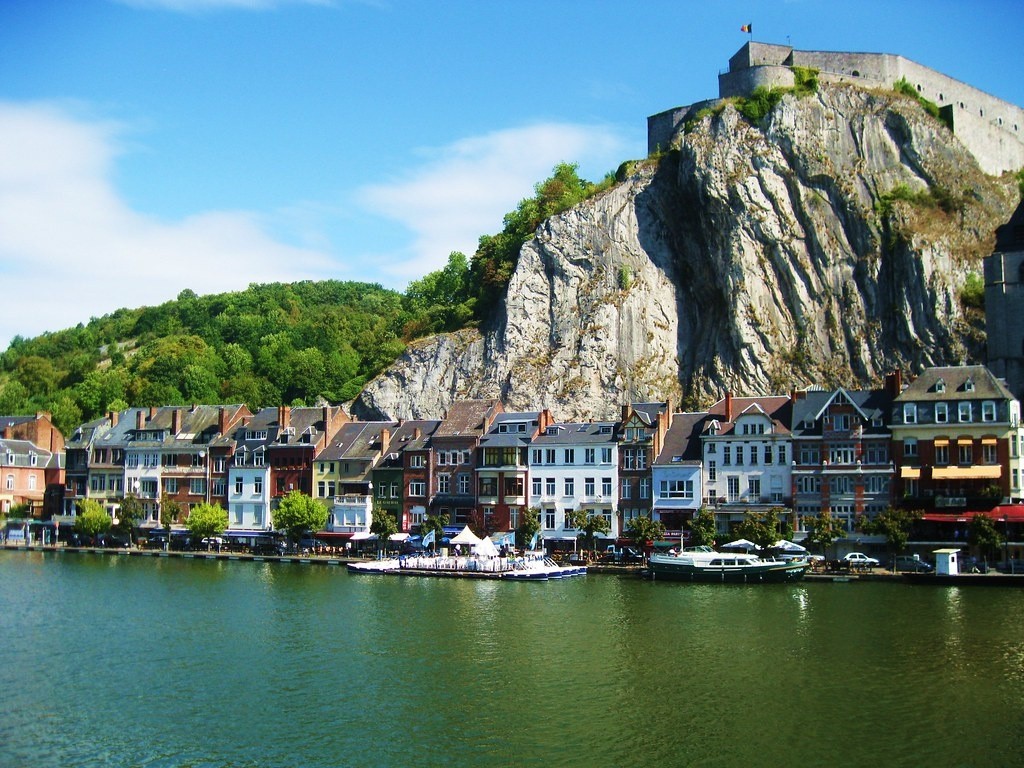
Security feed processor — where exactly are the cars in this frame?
[957,555,990,571]
[841,552,880,568]
[995,558,1024,573]
[886,555,934,573]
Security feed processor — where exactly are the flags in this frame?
[530,532,538,551]
[422,529,435,547]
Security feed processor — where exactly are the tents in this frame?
[349,532,412,551]
[473,535,500,560]
[449,525,482,556]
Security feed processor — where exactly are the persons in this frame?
[667,547,681,556]
[712,538,720,553]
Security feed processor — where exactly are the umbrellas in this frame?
[721,539,764,554]
[766,539,806,554]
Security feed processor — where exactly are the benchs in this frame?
[851,564,873,572]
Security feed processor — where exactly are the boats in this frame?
[648,527,810,584]
[347,549,589,582]
